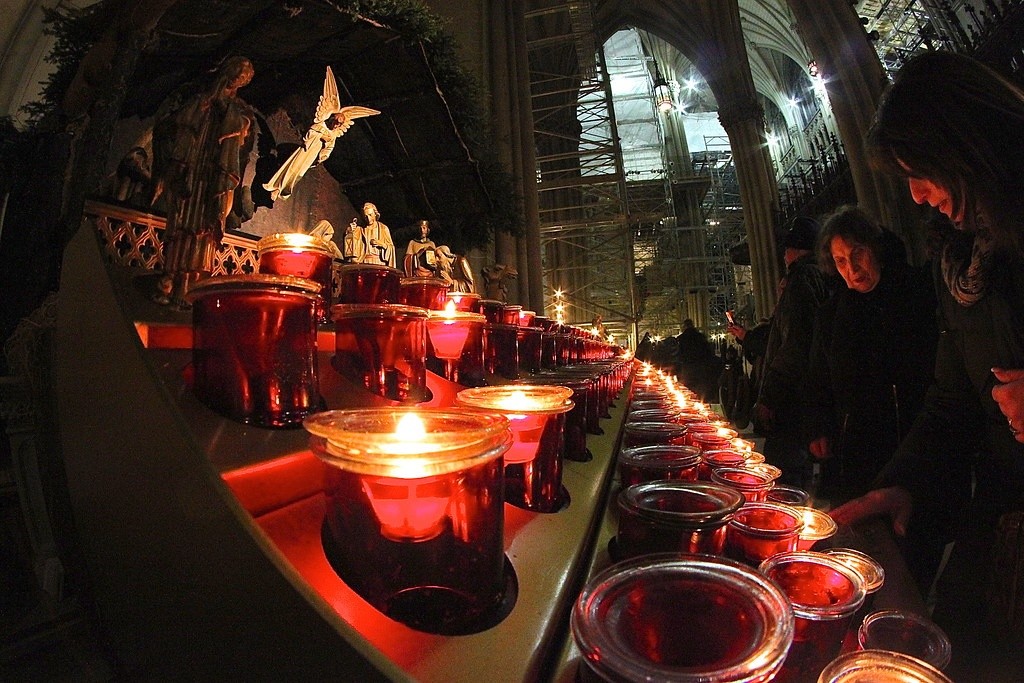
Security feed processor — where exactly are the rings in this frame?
[1009,417,1020,435]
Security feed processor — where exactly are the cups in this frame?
[183,230,955,683]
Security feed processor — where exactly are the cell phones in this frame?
[724,310,735,326]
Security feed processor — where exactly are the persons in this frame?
[431,245,475,293]
[305,220,344,260]
[701,51,1024,683]
[592,315,611,343]
[402,221,436,279]
[621,317,717,398]
[262,113,345,203]
[343,202,397,269]
[152,56,263,312]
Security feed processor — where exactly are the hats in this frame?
[783,216,824,249]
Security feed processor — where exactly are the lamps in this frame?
[645,31,672,112]
